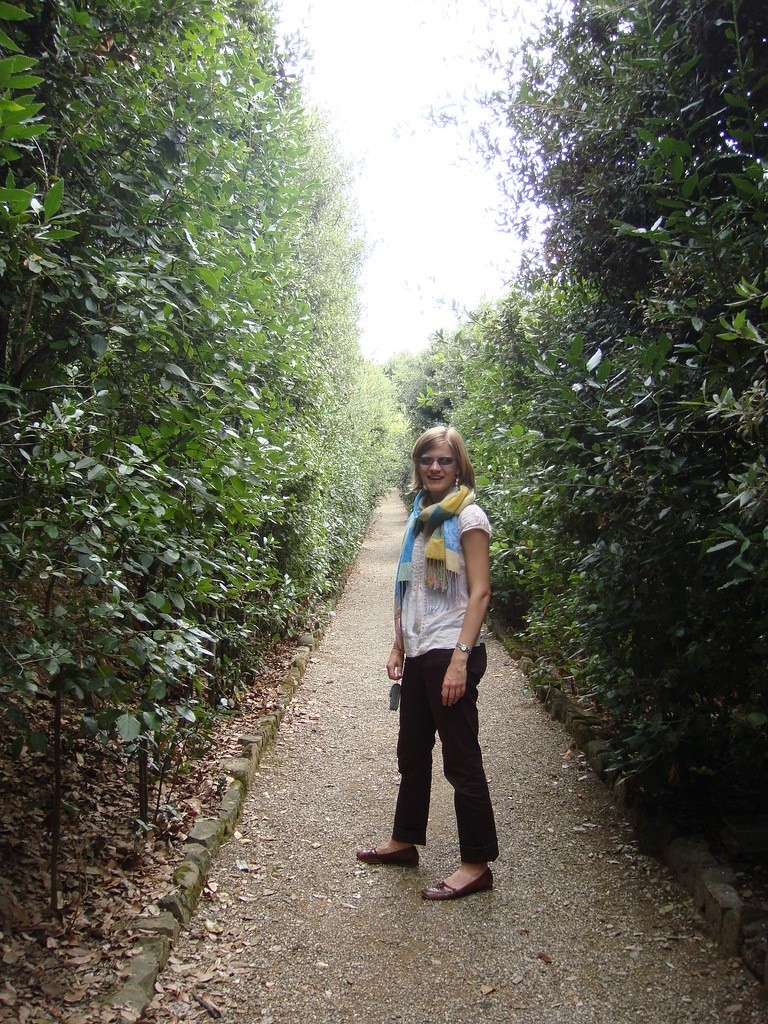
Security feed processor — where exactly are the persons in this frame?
[356,425,499,899]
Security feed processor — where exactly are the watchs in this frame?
[456,642,472,654]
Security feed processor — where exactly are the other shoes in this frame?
[421,867,493,900]
[356,846,419,868]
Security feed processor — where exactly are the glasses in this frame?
[416,457,457,466]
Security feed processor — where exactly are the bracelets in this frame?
[393,647,399,650]
